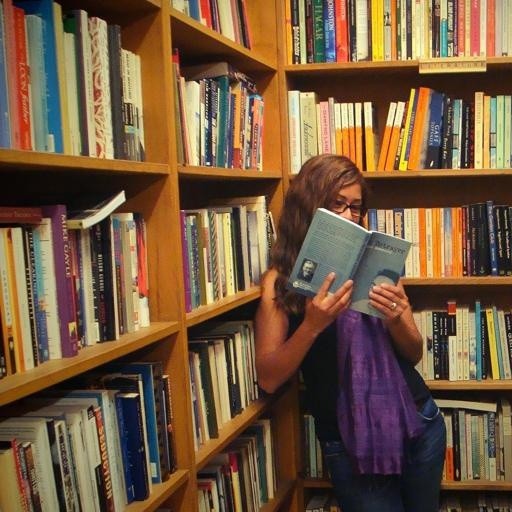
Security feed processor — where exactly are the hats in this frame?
[378,269,400,286]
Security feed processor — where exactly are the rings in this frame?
[390,302,397,310]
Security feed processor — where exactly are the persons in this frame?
[298,260,314,282]
[350,268,399,319]
[254,152,448,512]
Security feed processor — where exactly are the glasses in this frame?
[332,200,364,217]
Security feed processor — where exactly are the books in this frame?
[173,1,251,50]
[0,360,177,512]
[409,300,511,381]
[2,0,146,161]
[284,207,413,319]
[303,414,338,510]
[180,195,278,313]
[172,47,265,170]
[285,1,512,65]
[2,192,152,377]
[288,86,512,174]
[361,201,512,279]
[433,398,511,511]
[188,320,279,512]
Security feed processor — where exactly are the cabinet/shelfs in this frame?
[0,2,303,512]
[279,0,512,512]
